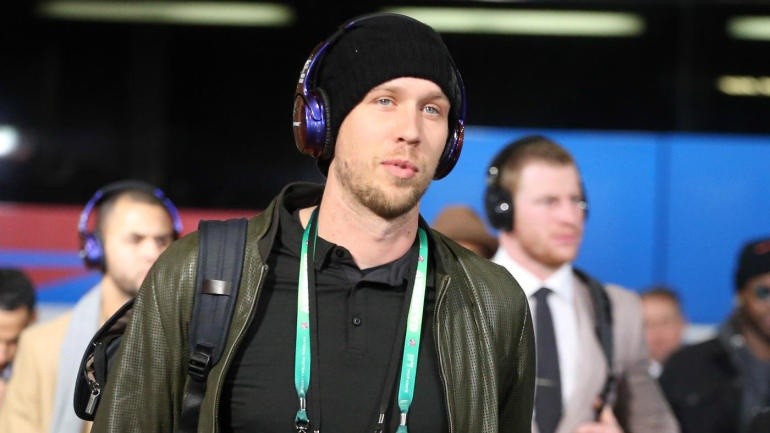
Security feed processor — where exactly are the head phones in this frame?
[76,181,181,270]
[484,137,589,229]
[292,10,469,180]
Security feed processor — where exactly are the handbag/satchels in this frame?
[71,217,247,422]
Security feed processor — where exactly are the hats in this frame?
[431,204,497,258]
[738,241,770,289]
[308,12,462,178]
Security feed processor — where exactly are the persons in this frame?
[0,179,182,433]
[90,12,536,433]
[0,269,36,400]
[660,238,770,433]
[487,136,680,433]
[640,290,681,360]
[430,207,499,259]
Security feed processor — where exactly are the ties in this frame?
[532,288,562,433]
